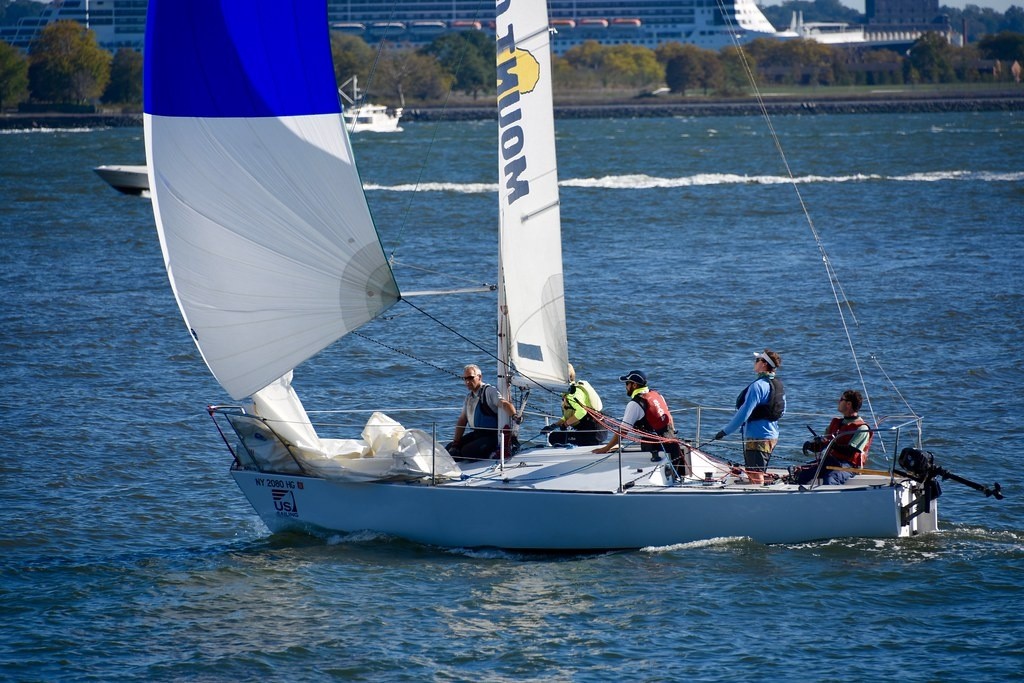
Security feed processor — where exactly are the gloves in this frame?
[560,422,568,431]
[512,413,524,425]
[448,442,460,455]
[540,423,558,435]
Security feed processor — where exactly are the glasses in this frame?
[840,397,848,402]
[756,358,763,362]
[462,375,476,381]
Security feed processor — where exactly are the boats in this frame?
[93,164,150,199]
[337,74,404,134]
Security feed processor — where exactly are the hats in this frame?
[619,370,647,385]
[753,351,777,369]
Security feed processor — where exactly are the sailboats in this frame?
[141,0,1006,544]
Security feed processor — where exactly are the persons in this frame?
[591,369,681,484]
[445,363,523,462]
[540,362,609,447]
[714,349,786,486]
[799,388,875,487]
